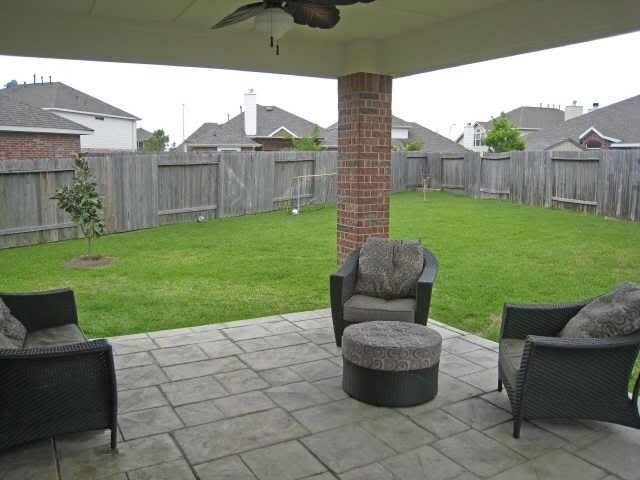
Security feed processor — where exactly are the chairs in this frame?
[0,286,117,450]
[499,296,640,438]
[330,240,439,347]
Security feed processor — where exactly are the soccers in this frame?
[198,216,205,222]
[292,209,298,215]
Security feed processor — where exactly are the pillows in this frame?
[353,236,424,300]
[0,296,28,349]
[557,281,640,337]
